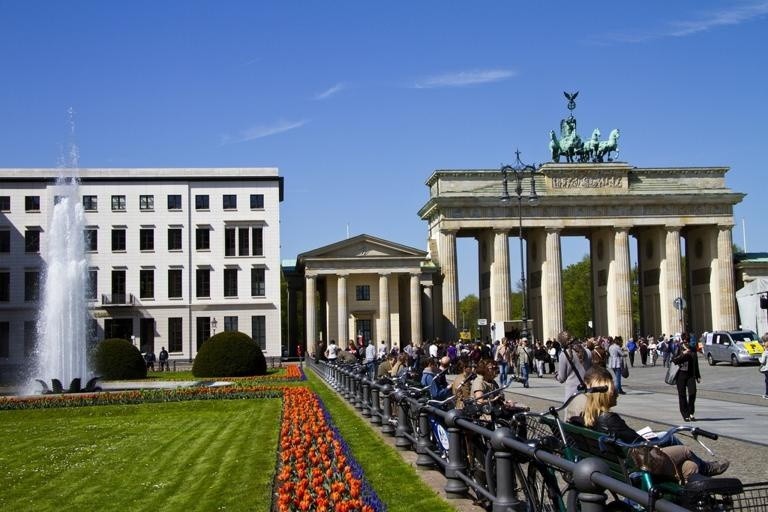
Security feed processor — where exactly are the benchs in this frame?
[551,422,685,510]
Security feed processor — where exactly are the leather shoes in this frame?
[684,414,695,421]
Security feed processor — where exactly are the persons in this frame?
[758,332,768,400]
[144,348,156,372]
[578,372,729,481]
[699,332,708,353]
[158,346,170,371]
[296,345,302,356]
[451,360,530,416]
[317,333,680,394]
[672,331,701,422]
[554,332,592,420]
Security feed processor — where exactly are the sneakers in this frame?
[703,459,729,476]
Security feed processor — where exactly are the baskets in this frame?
[713,481,768,512]
[516,413,575,452]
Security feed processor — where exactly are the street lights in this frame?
[211,317,218,336]
[500,148,546,338]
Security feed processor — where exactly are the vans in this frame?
[703,330,763,367]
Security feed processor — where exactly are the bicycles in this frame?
[331,353,768,512]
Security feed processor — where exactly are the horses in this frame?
[565,129,579,163]
[583,129,601,162]
[598,129,619,162]
[549,130,560,164]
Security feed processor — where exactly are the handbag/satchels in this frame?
[622,367,629,378]
[759,357,768,372]
[665,362,680,384]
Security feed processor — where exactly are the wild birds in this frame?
[563,91,579,102]
